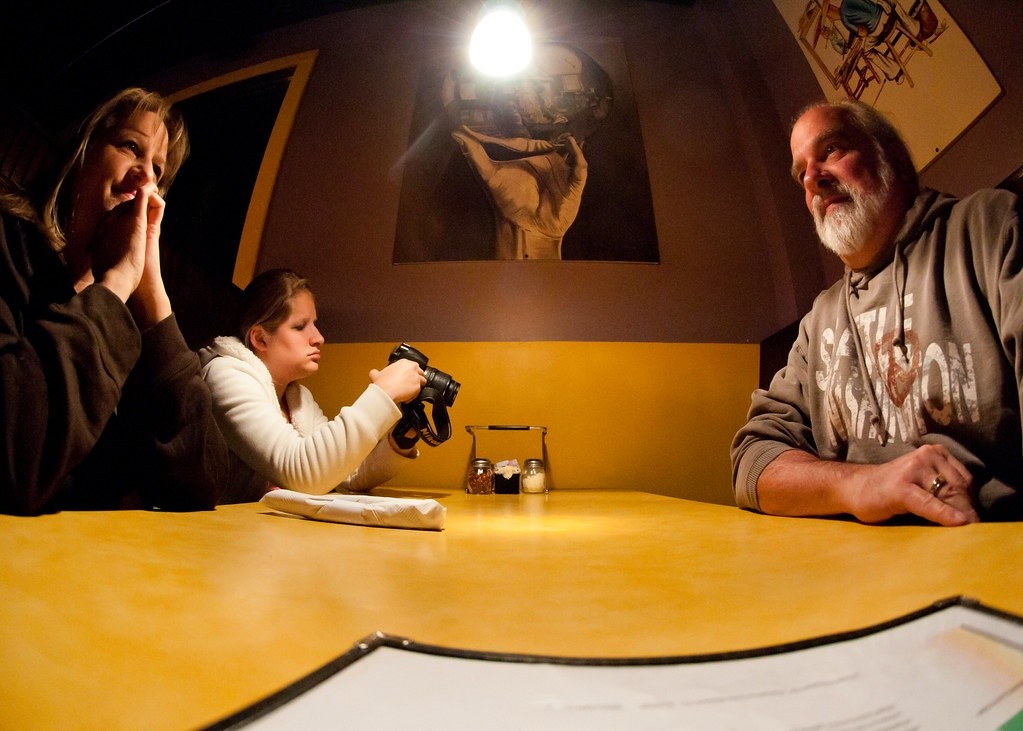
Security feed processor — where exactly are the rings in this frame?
[929,474,945,496]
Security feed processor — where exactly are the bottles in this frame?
[522,459,545,492]
[468,459,493,495]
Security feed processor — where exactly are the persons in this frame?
[730,101,1023,529]
[196,269,426,501]
[0,86,226,517]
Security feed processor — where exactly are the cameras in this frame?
[387,343,461,414]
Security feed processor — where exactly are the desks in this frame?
[0,490,1023,731]
